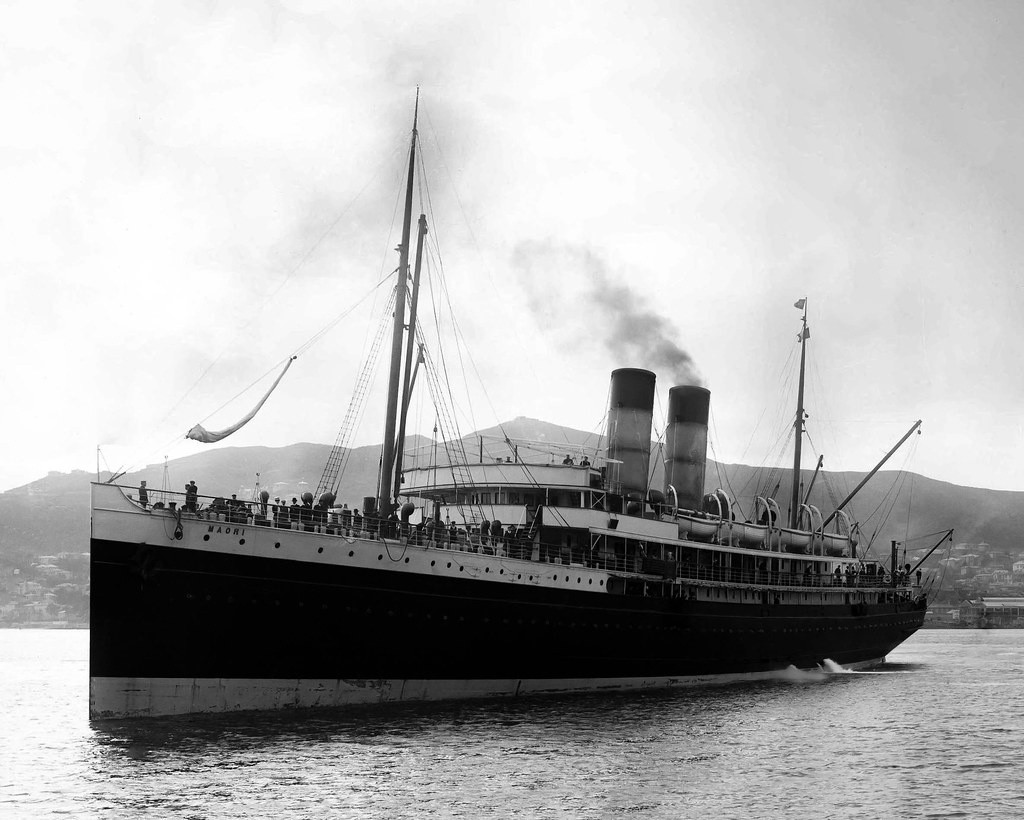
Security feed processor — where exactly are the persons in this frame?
[272,497,518,555]
[226,494,241,507]
[834,565,841,578]
[562,455,574,465]
[185,481,198,514]
[916,568,922,585]
[139,480,149,509]
[580,456,590,466]
[845,564,886,586]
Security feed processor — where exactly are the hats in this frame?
[451,521,456,523]
[353,509,359,511]
[292,498,297,501]
[275,497,280,501]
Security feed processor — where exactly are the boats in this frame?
[814,531,848,552]
[720,517,768,548]
[773,526,812,549]
[662,511,726,536]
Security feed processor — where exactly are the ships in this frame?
[85,85,956,720]
